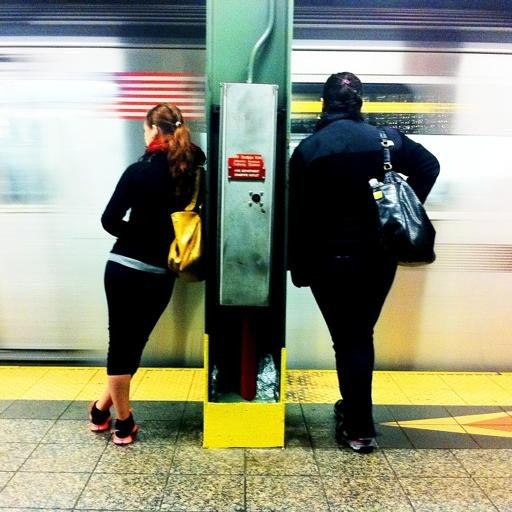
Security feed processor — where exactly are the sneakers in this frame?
[112,421,138,445]
[335,421,376,454]
[332,398,346,421]
[88,400,113,432]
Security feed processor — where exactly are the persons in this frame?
[88,100,208,444]
[290,70,440,455]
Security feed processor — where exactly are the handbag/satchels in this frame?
[370,127,437,268]
[167,168,205,285]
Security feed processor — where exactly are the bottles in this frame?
[367,177,385,201]
